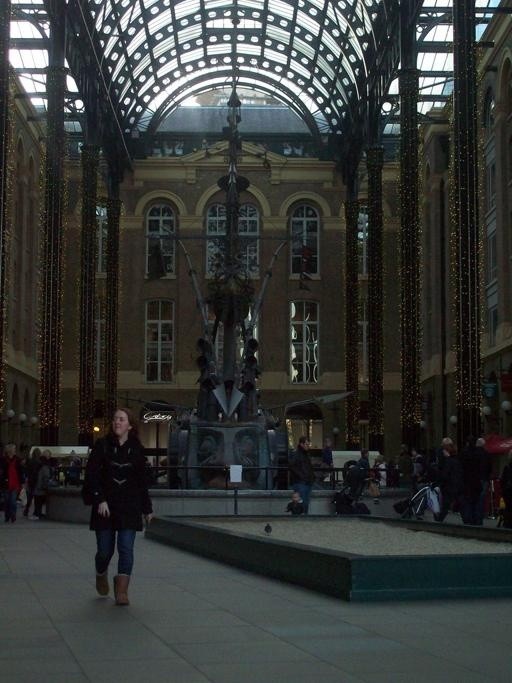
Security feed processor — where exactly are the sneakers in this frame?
[4,515,16,523]
[23,509,42,521]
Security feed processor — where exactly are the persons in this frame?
[82,407,153,605]
[0,444,81,523]
[287,437,512,529]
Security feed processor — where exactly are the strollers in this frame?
[330,467,372,513]
[393,484,431,521]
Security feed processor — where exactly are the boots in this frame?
[96,570,109,595]
[113,573,130,605]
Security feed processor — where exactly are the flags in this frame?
[300,248,312,291]
